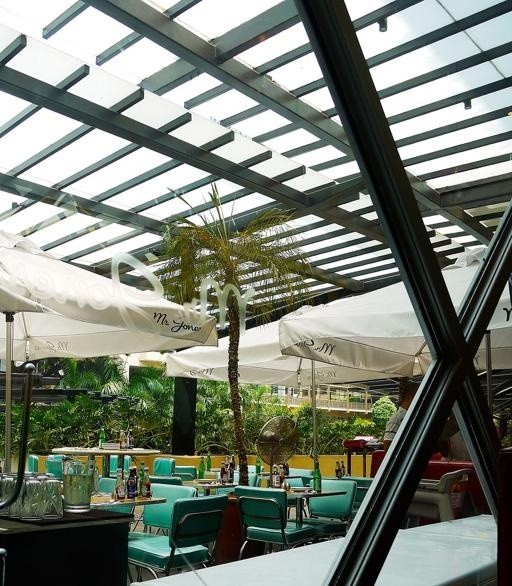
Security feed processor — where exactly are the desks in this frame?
[0,509,134,586]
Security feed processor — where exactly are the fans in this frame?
[256,416,300,487]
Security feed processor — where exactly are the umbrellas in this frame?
[279,245,512,411]
[1,246,219,473]
[166,313,404,470]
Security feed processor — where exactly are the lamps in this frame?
[463,98,472,109]
[378,17,387,31]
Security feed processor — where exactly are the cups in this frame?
[0,460,94,523]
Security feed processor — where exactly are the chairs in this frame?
[287,479,356,544]
[234,485,315,560]
[127,483,197,583]
[27,455,377,506]
[406,467,480,528]
[126,495,230,584]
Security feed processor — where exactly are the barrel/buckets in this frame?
[62,473,93,514]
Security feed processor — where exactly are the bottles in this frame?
[197,454,236,486]
[99,426,135,451]
[314,462,321,493]
[272,462,290,491]
[255,454,262,474]
[113,462,151,502]
[335,460,345,479]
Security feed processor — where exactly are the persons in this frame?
[383,383,420,451]
